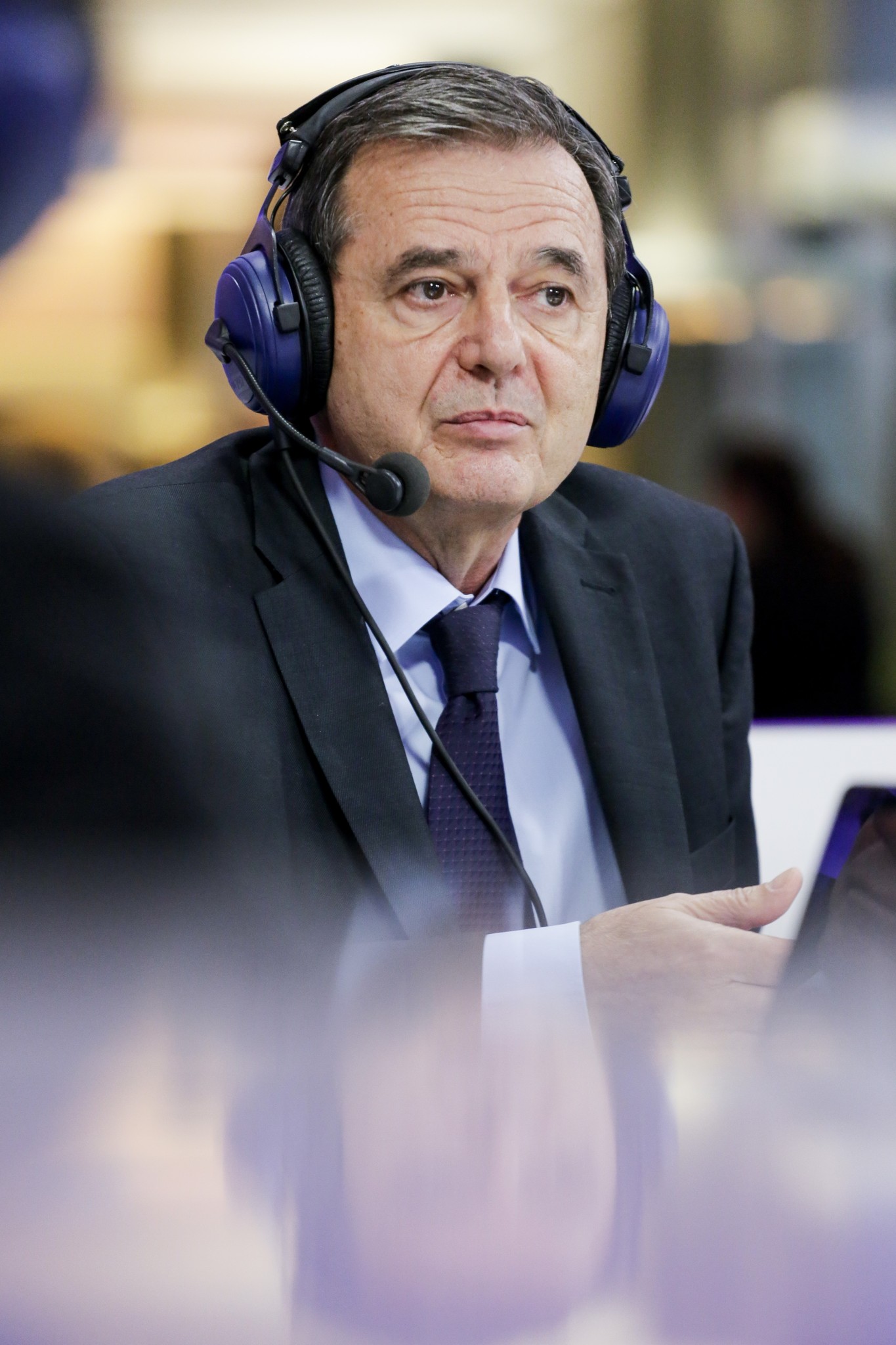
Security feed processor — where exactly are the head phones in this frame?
[211,61,668,447]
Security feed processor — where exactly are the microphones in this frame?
[205,318,429,516]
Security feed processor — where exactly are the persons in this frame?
[706,439,880,722]
[0,61,804,1345]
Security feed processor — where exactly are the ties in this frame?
[415,593,523,937]
